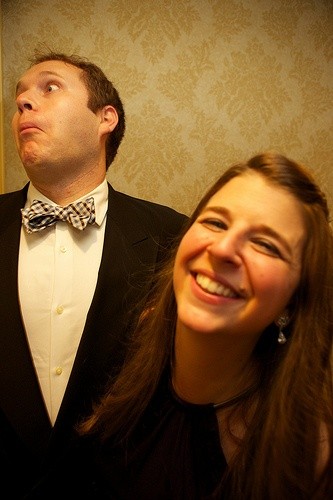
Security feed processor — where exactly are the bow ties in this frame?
[22,200,96,232]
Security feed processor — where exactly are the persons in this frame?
[75,153,333,500]
[0,54,192,500]
